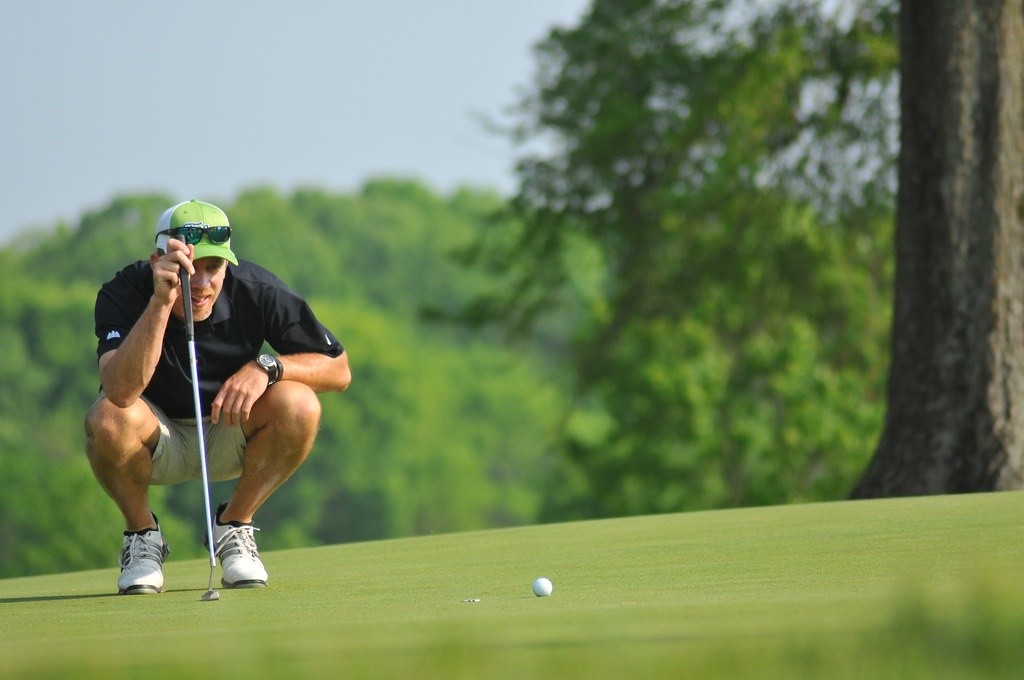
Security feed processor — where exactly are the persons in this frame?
[84,199,351,595]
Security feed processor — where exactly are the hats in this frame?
[155,200,238,267]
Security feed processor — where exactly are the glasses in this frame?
[156,226,233,247]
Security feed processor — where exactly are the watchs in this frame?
[255,354,278,386]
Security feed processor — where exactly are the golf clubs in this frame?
[174,231,221,603]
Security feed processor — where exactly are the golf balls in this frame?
[532,577,553,596]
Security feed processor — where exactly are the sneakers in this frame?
[204,503,268,589]
[118,513,170,594]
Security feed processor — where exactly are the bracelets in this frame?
[274,358,283,382]
